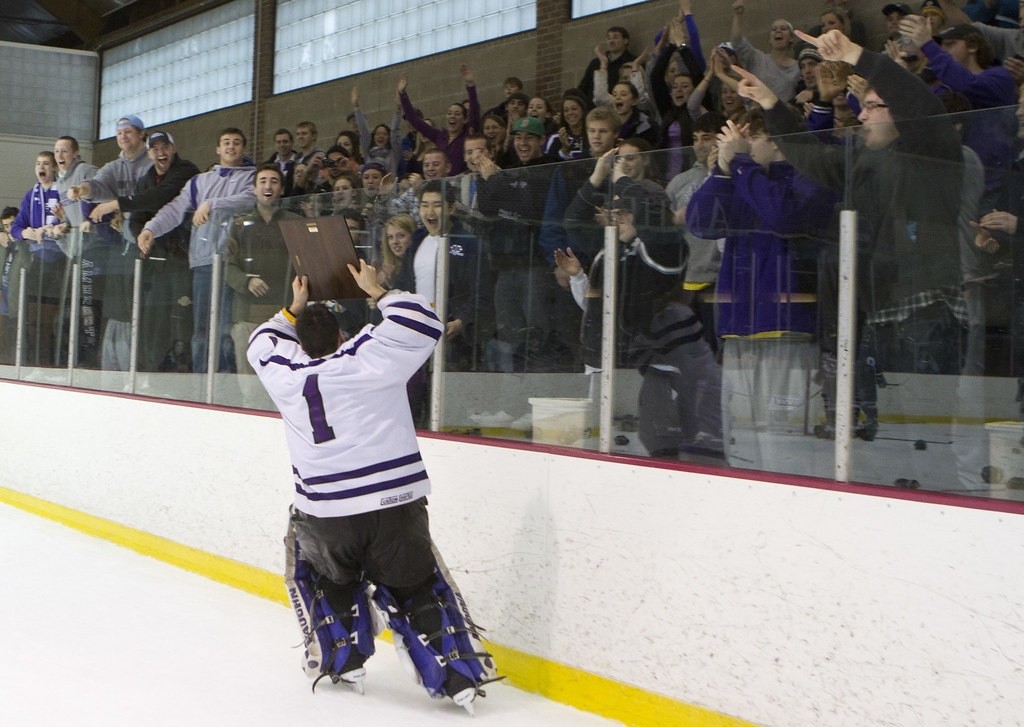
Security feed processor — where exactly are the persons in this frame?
[0,0,1024,466]
[246,258,498,715]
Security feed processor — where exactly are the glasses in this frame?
[861,102,888,113]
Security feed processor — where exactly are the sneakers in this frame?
[445,666,480,715]
[340,649,367,694]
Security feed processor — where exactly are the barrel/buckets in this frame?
[528,397,591,450]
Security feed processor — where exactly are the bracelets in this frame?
[704,79,712,83]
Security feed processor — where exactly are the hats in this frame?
[797,47,823,63]
[117,115,148,142]
[717,43,733,50]
[883,3,912,15]
[933,25,980,40]
[620,62,645,77]
[510,117,544,137]
[564,88,584,98]
[146,131,175,150]
[922,0,945,17]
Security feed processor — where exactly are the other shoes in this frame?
[813,421,837,441]
[857,420,878,440]
